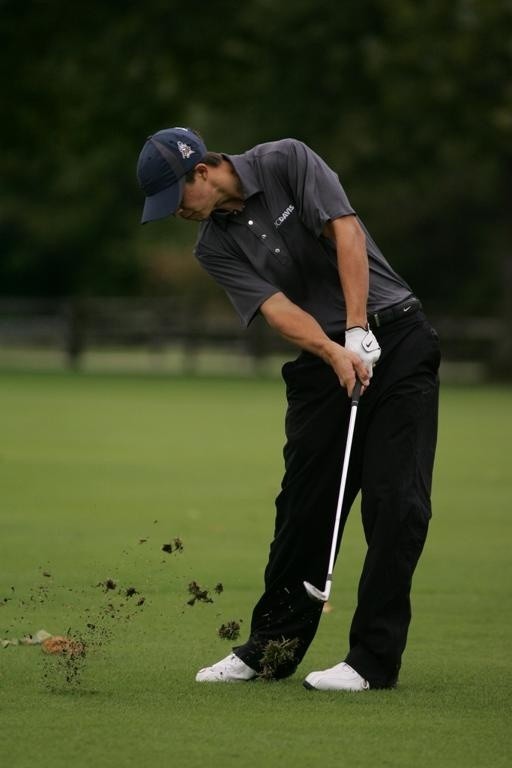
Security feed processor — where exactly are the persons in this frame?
[130,121,444,694]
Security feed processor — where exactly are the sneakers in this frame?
[195,650,261,685]
[303,661,370,693]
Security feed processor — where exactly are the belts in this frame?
[328,295,422,344]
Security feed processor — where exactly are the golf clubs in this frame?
[303,373,362,601]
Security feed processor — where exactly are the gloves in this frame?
[345,323,382,381]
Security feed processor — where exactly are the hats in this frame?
[137,127,207,226]
